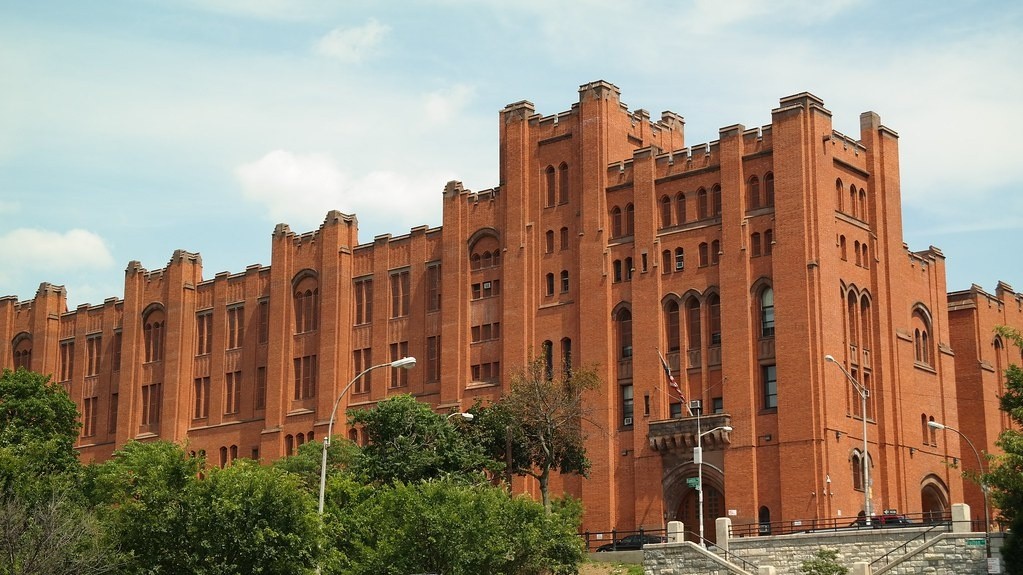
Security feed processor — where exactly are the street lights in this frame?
[928,422,991,557]
[698,426,733,547]
[825,355,871,525]
[315,356,416,575]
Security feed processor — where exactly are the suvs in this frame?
[850,515,912,527]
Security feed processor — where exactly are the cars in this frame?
[596,535,669,552]
